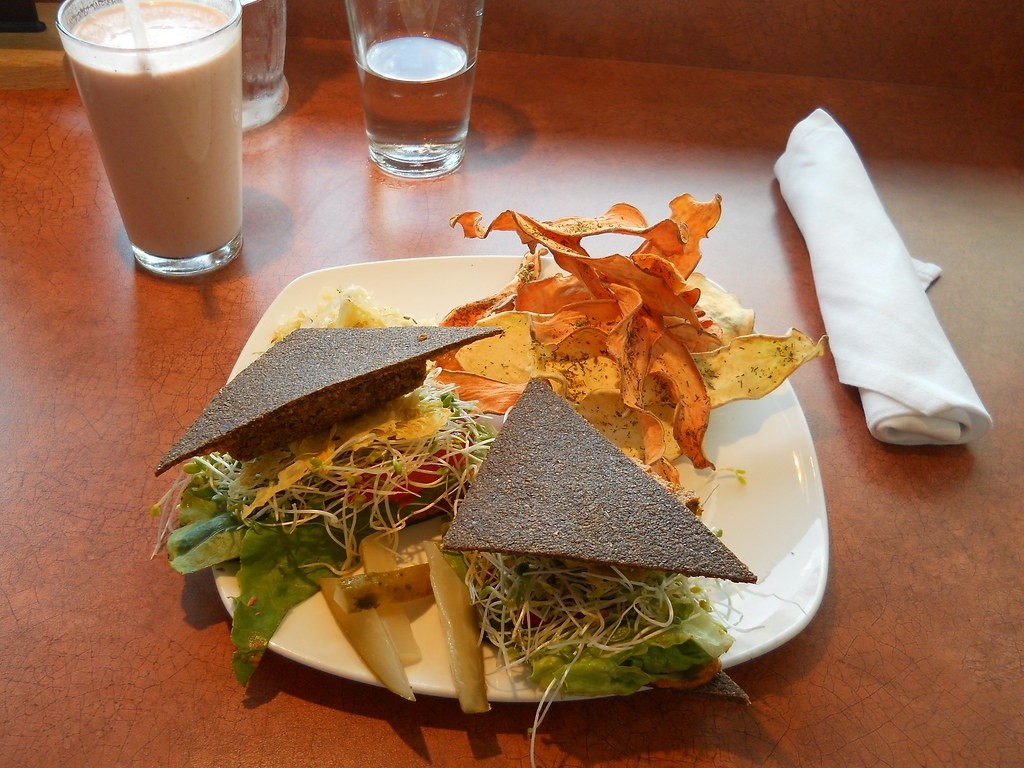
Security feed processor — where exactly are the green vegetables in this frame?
[144,392,747,767]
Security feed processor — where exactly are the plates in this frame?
[210,255,831,700]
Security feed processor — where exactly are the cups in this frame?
[345,0,484,180]
[231,0,290,133]
[53,0,243,277]
